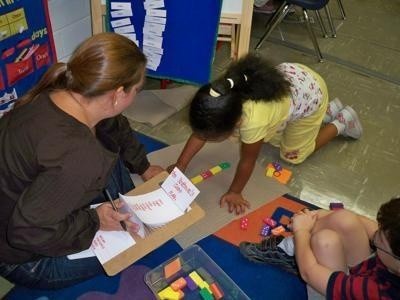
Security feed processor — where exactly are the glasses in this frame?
[369,231,399,261]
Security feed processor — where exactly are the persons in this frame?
[238,197,399,300]
[1,32,165,290]
[167,53,363,216]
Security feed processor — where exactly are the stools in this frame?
[253,0,347,63]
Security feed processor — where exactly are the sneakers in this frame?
[240,235,298,275]
[322,97,363,139]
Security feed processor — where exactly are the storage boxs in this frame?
[142,243,253,300]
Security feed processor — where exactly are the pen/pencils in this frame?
[14,44,40,64]
[105,189,128,232]
